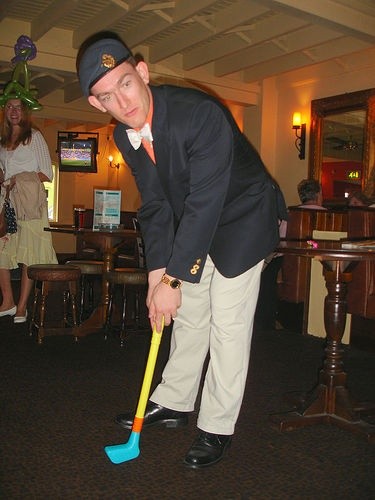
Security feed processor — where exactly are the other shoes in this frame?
[13,309,28,323]
[0,306,17,317]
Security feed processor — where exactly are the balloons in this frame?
[0,35,43,112]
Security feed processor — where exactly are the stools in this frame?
[62,260,104,329]
[27,263,83,344]
[105,267,149,350]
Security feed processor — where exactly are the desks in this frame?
[44,224,152,336]
[263,231,375,444]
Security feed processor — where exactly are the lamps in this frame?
[292,111,307,160]
[108,154,121,169]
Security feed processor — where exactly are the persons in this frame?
[0,93,58,323]
[76,39,289,471]
[347,192,375,207]
[268,178,328,271]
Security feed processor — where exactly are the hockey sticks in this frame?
[105,315,164,464]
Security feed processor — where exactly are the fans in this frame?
[325,135,363,150]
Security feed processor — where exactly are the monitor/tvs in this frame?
[58,137,97,173]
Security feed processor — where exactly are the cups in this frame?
[72,203,85,229]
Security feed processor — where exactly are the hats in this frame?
[77,38,132,98]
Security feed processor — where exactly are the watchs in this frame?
[162,276,181,289]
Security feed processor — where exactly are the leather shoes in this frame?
[114,398,189,431]
[183,429,235,468]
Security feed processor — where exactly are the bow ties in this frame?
[126,123,154,151]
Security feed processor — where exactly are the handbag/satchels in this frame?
[0,187,11,238]
